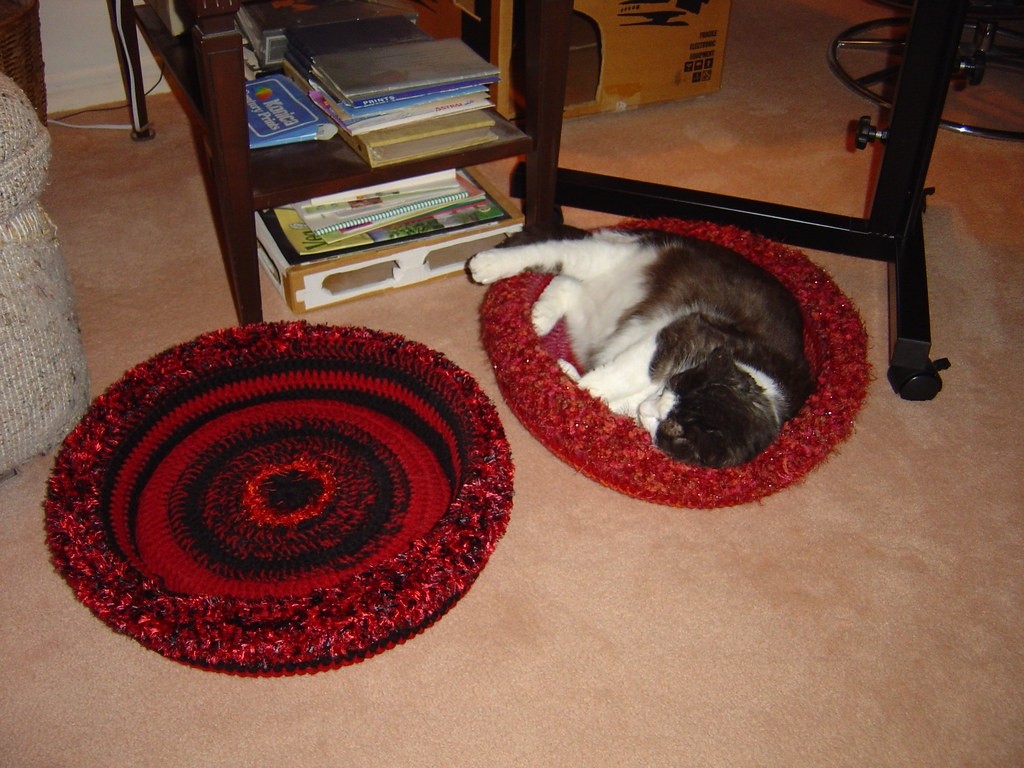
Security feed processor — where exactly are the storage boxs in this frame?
[461,1,729,121]
[255,165,526,317]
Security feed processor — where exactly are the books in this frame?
[256,168,512,267]
[308,38,503,168]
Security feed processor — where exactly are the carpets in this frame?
[43,318,516,679]
[478,216,878,508]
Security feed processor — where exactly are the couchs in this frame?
[1,70,94,485]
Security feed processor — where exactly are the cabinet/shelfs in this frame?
[131,0,572,329]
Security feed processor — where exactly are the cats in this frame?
[466,228,814,468]
